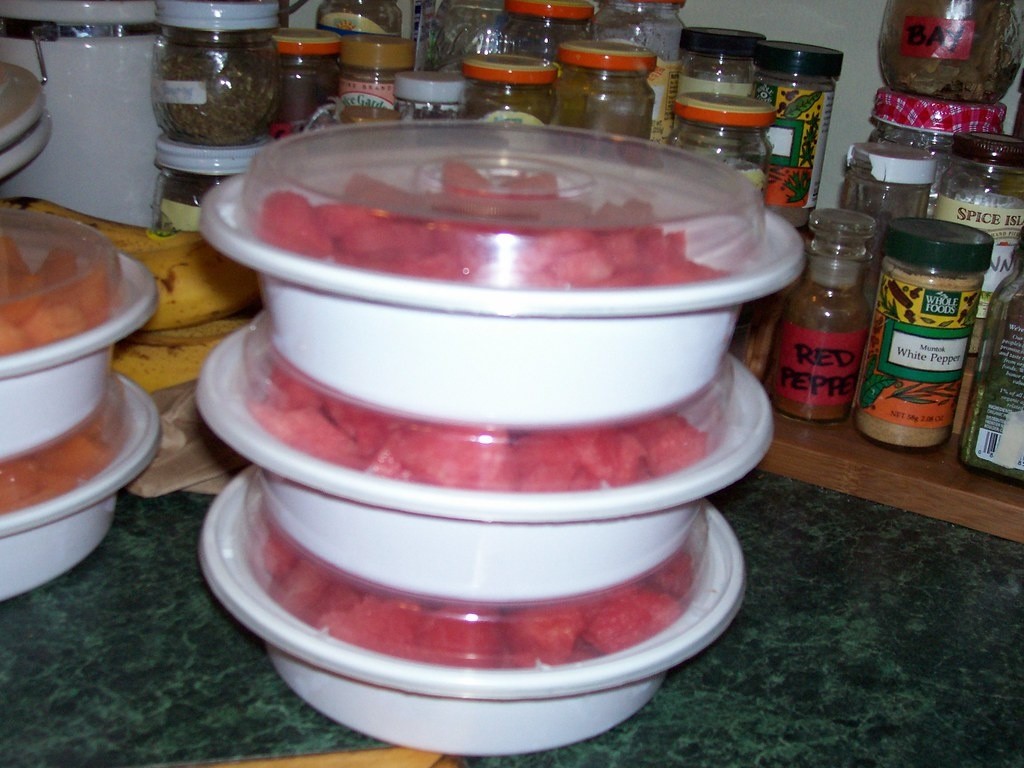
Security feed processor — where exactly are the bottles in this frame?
[197,118,807,426]
[774,207,875,421]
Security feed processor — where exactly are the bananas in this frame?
[0,197,263,394]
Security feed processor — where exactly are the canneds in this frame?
[152,0,1024,444]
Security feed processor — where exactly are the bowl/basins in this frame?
[0,369,163,603]
[0,206,159,463]
[197,313,775,607]
[197,465,747,755]
